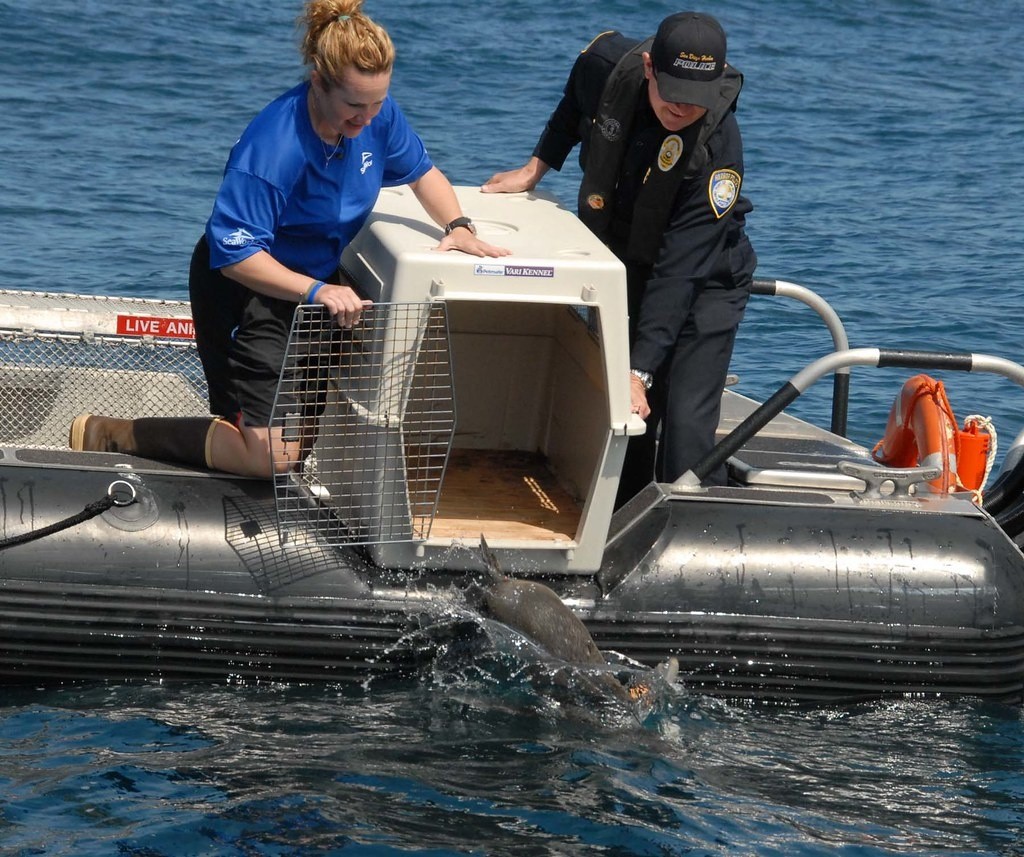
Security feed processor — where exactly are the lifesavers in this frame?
[880,373,958,492]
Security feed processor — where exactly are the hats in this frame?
[651,11,727,111]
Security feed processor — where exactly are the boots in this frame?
[68,412,222,469]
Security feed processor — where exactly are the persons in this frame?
[68,1,514,481]
[482,10,759,516]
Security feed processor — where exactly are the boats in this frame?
[1,272,1023,709]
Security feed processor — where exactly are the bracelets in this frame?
[303,281,326,304]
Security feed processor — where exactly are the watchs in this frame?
[445,216,476,234]
[631,369,653,389]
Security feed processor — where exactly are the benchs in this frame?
[656,388,880,492]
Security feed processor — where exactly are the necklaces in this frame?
[312,91,343,170]
